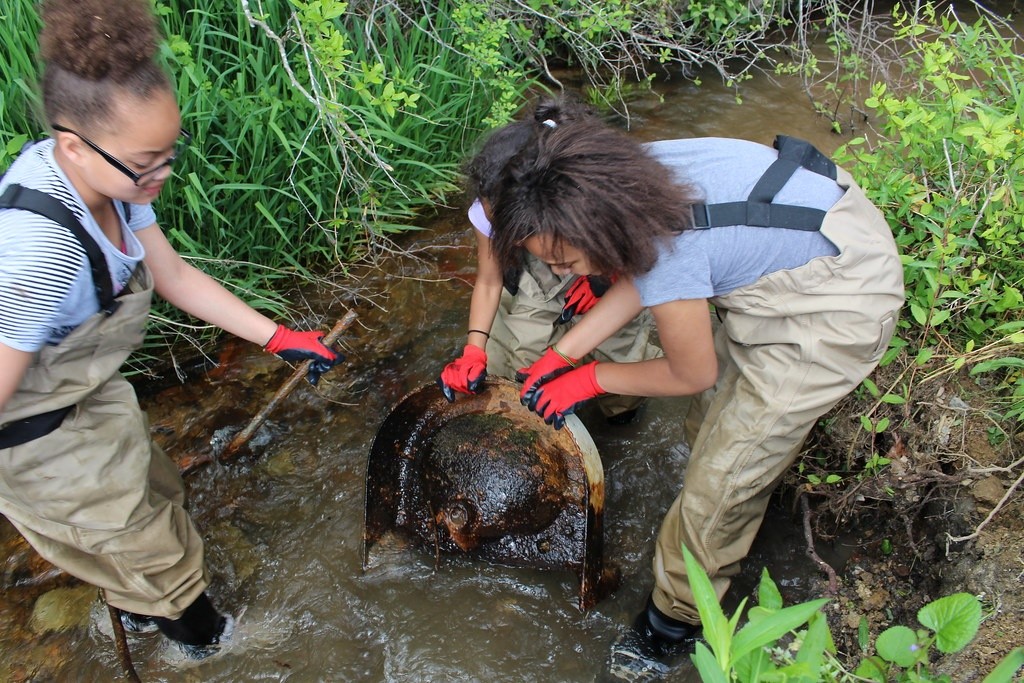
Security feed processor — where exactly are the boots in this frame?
[156,592,225,660]
[615,591,699,675]
[119,609,155,634]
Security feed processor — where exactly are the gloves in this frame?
[514,346,580,407]
[437,343,487,403]
[563,270,622,315]
[528,359,607,430]
[261,323,345,386]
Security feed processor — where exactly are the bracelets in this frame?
[552,342,579,369]
[466,328,490,339]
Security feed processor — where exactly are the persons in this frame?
[0,0,346,661]
[435,91,906,683]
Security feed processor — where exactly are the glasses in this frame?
[50,120,193,188]
